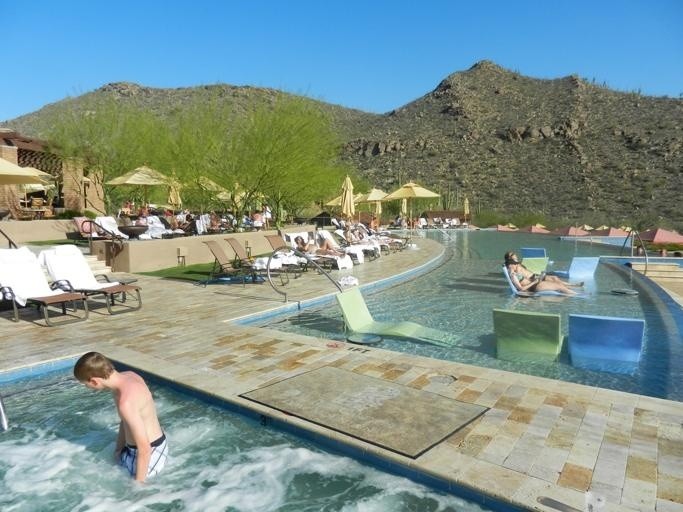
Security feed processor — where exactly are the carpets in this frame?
[239,362,493,460]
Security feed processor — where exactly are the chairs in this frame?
[0,248,87,325]
[501,246,601,300]
[337,289,462,350]
[39,246,141,314]
[565,314,647,376]
[492,306,564,367]
[6,186,50,222]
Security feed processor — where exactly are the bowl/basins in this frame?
[118,224,149,240]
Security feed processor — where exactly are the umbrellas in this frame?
[105,160,172,207]
[19,166,50,208]
[0,158,46,186]
[167,174,443,247]
[463,199,470,225]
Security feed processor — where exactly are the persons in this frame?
[504,260,579,296]
[293,235,347,259]
[75,352,167,485]
[661,248,670,255]
[637,244,644,254]
[111,200,271,238]
[504,252,586,287]
